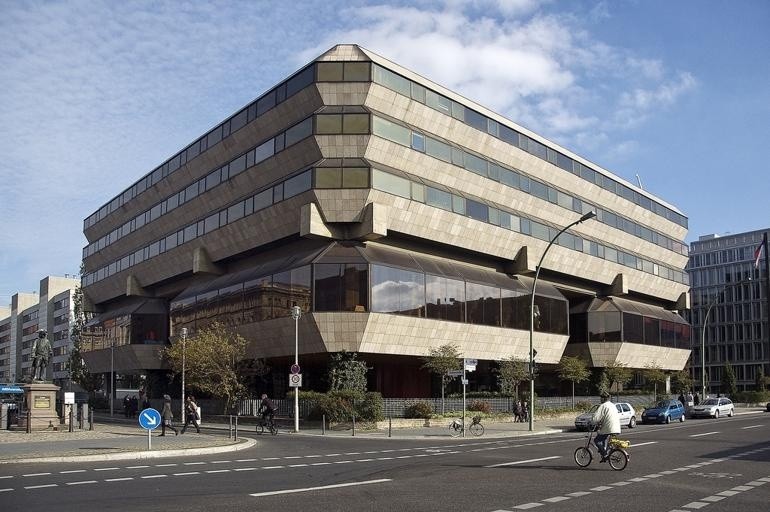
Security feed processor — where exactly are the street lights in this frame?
[703,278,752,398]
[181,328,187,424]
[529,211,597,429]
[292,305,301,432]
[110,342,115,417]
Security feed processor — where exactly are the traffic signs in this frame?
[462,380,468,385]
[447,370,464,376]
[466,360,478,372]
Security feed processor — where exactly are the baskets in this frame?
[473,416,481,424]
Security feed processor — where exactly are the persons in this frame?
[677,392,685,407]
[131,395,139,419]
[522,400,530,422]
[693,390,701,406]
[158,393,179,438]
[513,400,526,422]
[686,391,694,407]
[141,396,152,410]
[257,393,280,428]
[180,395,202,436]
[121,394,132,420]
[30,328,54,380]
[587,390,622,463]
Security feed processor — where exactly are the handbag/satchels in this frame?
[195,412,200,420]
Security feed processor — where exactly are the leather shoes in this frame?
[598,449,605,452]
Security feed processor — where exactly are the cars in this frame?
[575,403,637,428]
[641,399,685,423]
[690,398,735,419]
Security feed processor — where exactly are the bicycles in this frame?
[256,412,278,436]
[574,422,632,470]
[449,416,485,437]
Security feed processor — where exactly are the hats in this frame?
[164,395,171,400]
[600,391,611,399]
